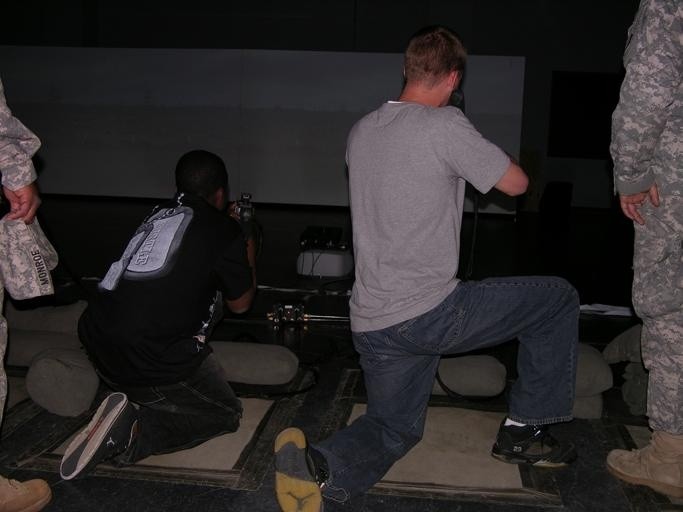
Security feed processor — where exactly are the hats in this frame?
[1,215,60,300]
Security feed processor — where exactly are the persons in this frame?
[0,80,59,512]
[274,28,579,512]
[59,150,258,480]
[605,0,683,506]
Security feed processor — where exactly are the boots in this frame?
[606,429,682,501]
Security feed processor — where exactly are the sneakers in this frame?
[0,477,51,511]
[274,428,325,512]
[61,393,138,481]
[490,418,576,470]
